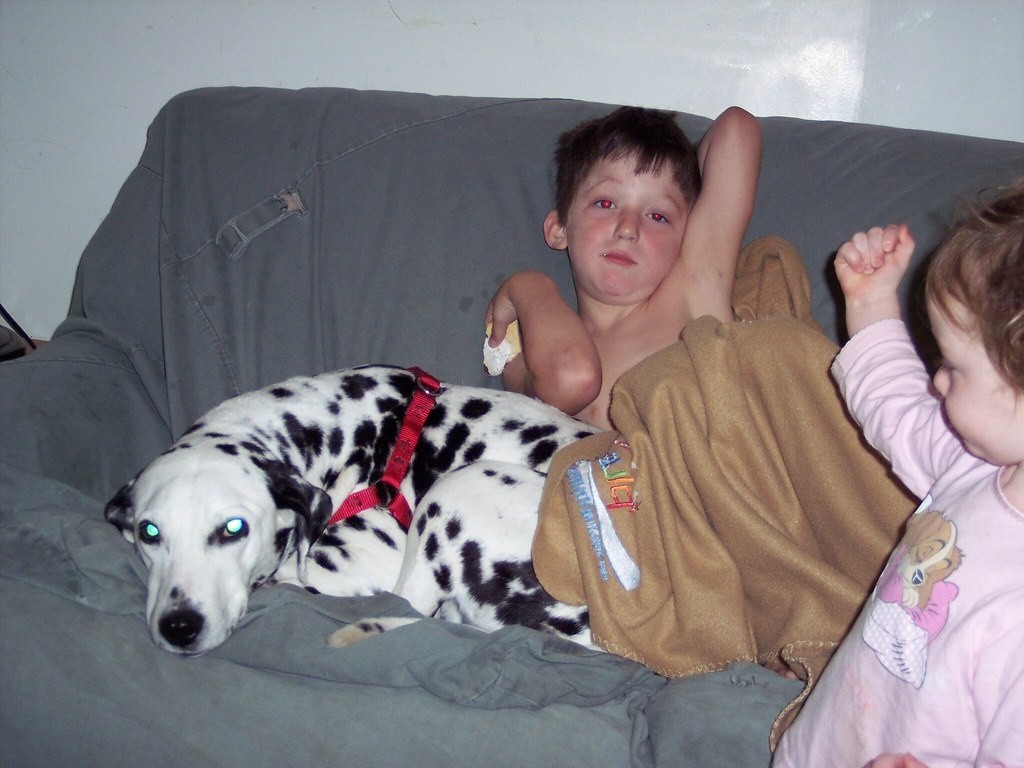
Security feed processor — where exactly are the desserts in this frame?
[482,318,523,376]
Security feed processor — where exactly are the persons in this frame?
[772,178,1023,767]
[484,107,764,432]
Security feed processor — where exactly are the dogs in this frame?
[101,363,611,657]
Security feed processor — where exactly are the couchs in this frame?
[0,85,1024,768]
